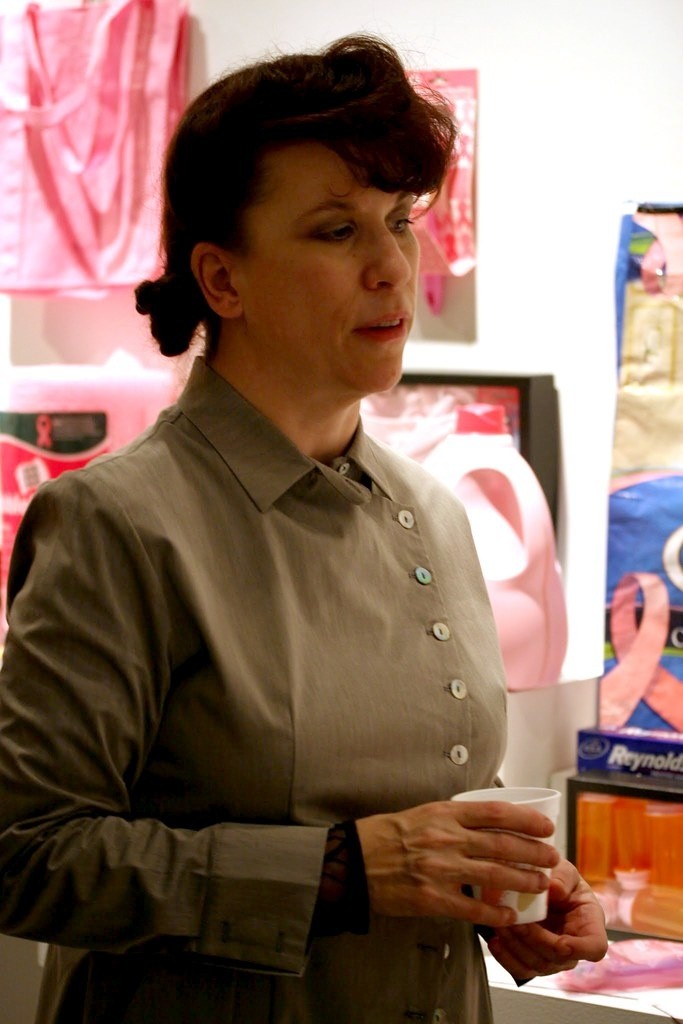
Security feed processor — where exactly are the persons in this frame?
[0,29,610,1024]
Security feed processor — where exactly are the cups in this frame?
[449,787,560,923]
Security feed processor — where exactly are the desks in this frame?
[484,931,683,1024]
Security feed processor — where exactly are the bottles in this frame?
[424,401,568,694]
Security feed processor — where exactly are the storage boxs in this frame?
[562,769,683,944]
